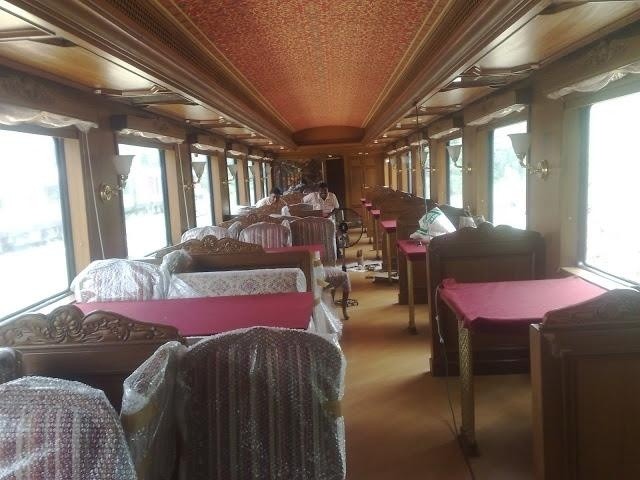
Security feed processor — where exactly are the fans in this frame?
[325,207,364,319]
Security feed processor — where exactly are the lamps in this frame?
[389,132,552,182]
[100,155,272,204]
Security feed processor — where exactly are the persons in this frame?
[301,183,339,218]
[256,186,288,214]
